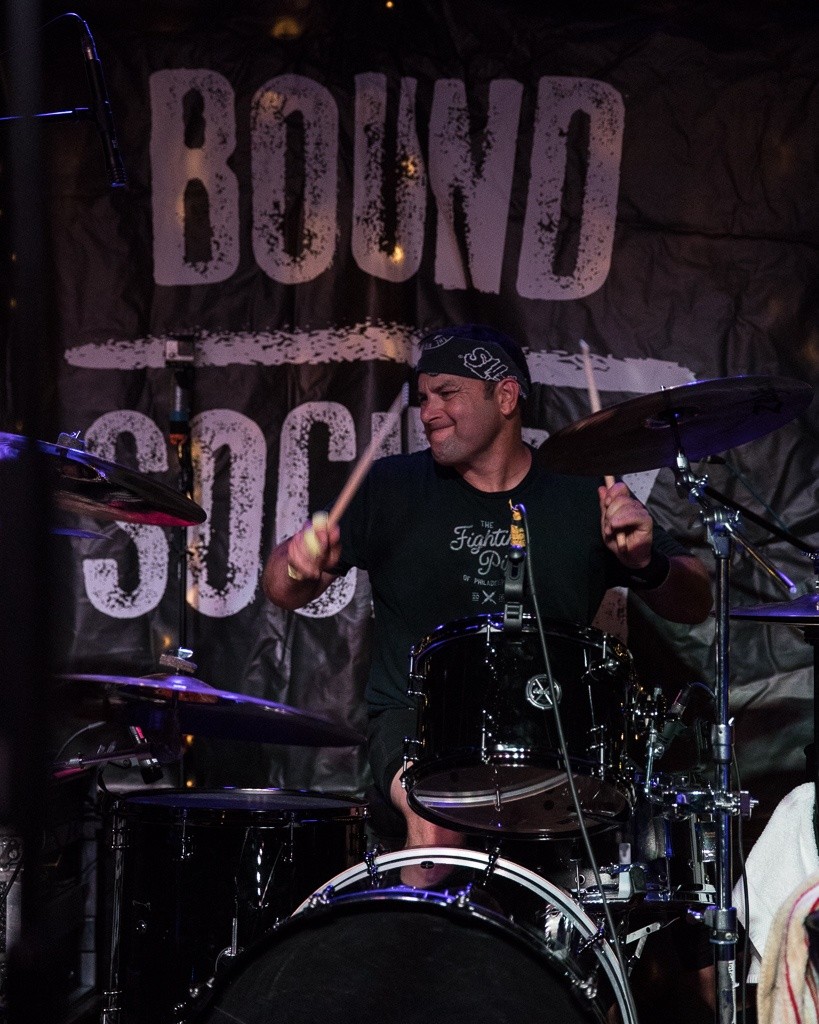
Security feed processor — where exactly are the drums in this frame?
[198,848,617,1024]
[405,609,715,909]
[82,781,378,996]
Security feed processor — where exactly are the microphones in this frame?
[164,336,199,446]
[652,684,695,758]
[511,509,526,551]
[77,20,128,188]
[691,454,727,465]
[125,720,164,784]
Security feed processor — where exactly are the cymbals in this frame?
[45,667,369,751]
[709,591,819,626]
[534,372,816,477]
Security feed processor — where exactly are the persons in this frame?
[263,320,757,1023]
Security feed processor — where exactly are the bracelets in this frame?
[623,548,672,592]
